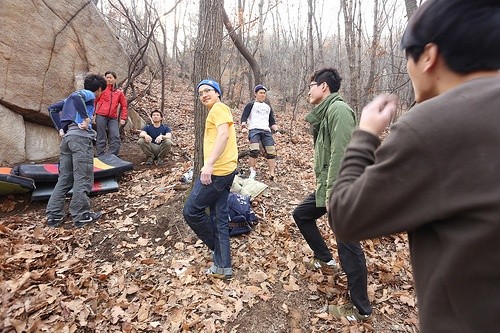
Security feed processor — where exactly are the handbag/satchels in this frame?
[227,191,259,237]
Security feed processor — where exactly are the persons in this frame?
[183,80,238,278]
[329,0,500,333]
[241,85,281,182]
[45,75,106,225]
[293,67,371,321]
[137,110,172,165]
[95,71,127,156]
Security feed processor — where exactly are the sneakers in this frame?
[328,303,373,324]
[303,252,338,274]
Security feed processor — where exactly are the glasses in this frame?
[308,83,319,90]
[197,88,216,96]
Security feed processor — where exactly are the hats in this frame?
[197,78,221,96]
[254,83,267,92]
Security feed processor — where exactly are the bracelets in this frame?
[242,125,246,128]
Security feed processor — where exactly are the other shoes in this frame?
[204,263,232,280]
[146,156,153,164]
[48,213,67,224]
[210,249,216,268]
[73,212,102,227]
[157,156,163,164]
